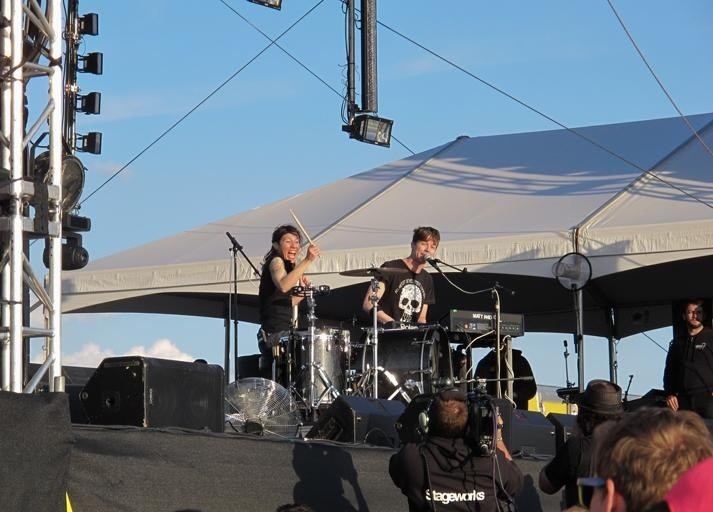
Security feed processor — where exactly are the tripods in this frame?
[266,293,341,424]
[352,284,411,405]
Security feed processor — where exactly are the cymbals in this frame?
[336,266,409,280]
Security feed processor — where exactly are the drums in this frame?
[270,327,352,410]
[351,327,379,348]
[362,325,453,407]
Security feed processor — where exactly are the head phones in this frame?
[418,387,459,434]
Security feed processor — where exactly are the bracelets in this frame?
[496,437,503,441]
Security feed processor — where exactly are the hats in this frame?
[577,379,624,415]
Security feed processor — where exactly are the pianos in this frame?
[400,306,523,345]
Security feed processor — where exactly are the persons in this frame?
[551,403,713,512]
[258,223,320,334]
[538,376,626,508]
[476,346,538,410]
[387,397,525,511]
[661,298,712,437]
[360,224,441,328]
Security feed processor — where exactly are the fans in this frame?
[223,377,302,437]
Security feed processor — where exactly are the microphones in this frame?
[421,253,445,276]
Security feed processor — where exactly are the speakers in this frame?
[79,356,226,435]
[547,413,584,454]
[511,410,556,454]
[308,395,409,447]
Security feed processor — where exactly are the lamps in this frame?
[42,214,91,270]
[61,0,103,155]
[340,0,394,148]
